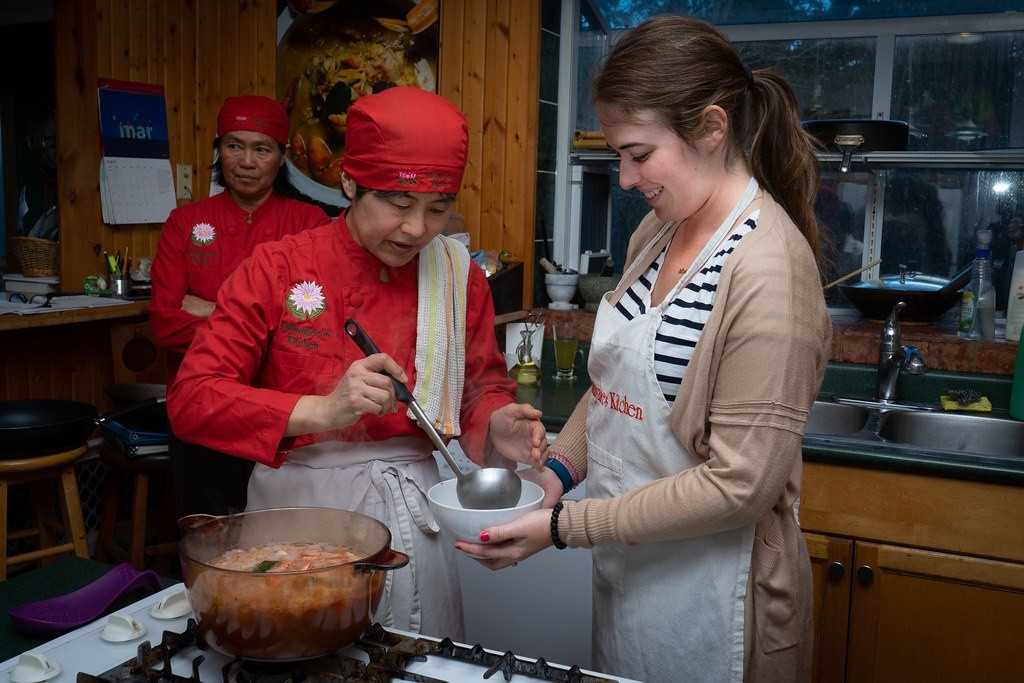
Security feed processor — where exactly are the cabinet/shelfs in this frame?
[795,461,1024,683]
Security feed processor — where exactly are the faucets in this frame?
[877,301,926,403]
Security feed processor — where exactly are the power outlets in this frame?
[176,163,193,200]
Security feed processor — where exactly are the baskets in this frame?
[8,236,60,276]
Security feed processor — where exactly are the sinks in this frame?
[870,396,1024,466]
[803,390,871,443]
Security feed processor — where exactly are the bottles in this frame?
[508,330,543,386]
[956,248,997,343]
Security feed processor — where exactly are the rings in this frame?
[512,562,519,567]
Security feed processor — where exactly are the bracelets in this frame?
[543,456,574,494]
[550,501,567,550]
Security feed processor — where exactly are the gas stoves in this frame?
[0,581,646,682]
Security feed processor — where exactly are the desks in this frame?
[0,291,150,332]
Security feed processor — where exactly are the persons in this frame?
[454,14,833,683]
[149,95,333,516]
[165,87,548,648]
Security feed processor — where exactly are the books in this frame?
[5,279,59,294]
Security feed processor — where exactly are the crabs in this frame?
[277,0,439,187]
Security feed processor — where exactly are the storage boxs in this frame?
[9,237,59,276]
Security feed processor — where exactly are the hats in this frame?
[342,88,468,194]
[217,96,288,146]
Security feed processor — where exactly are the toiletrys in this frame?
[955,228,997,342]
[1006,249,1024,341]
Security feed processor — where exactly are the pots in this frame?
[836,258,977,323]
[174,507,411,660]
[1,380,166,463]
[798,118,908,175]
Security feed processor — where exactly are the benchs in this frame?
[94,441,177,571]
[0,400,98,581]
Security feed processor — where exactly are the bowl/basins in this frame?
[275,0,437,208]
[427,476,546,559]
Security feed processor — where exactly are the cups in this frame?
[109,274,128,299]
[552,323,580,382]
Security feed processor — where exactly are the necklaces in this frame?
[680,216,690,275]
